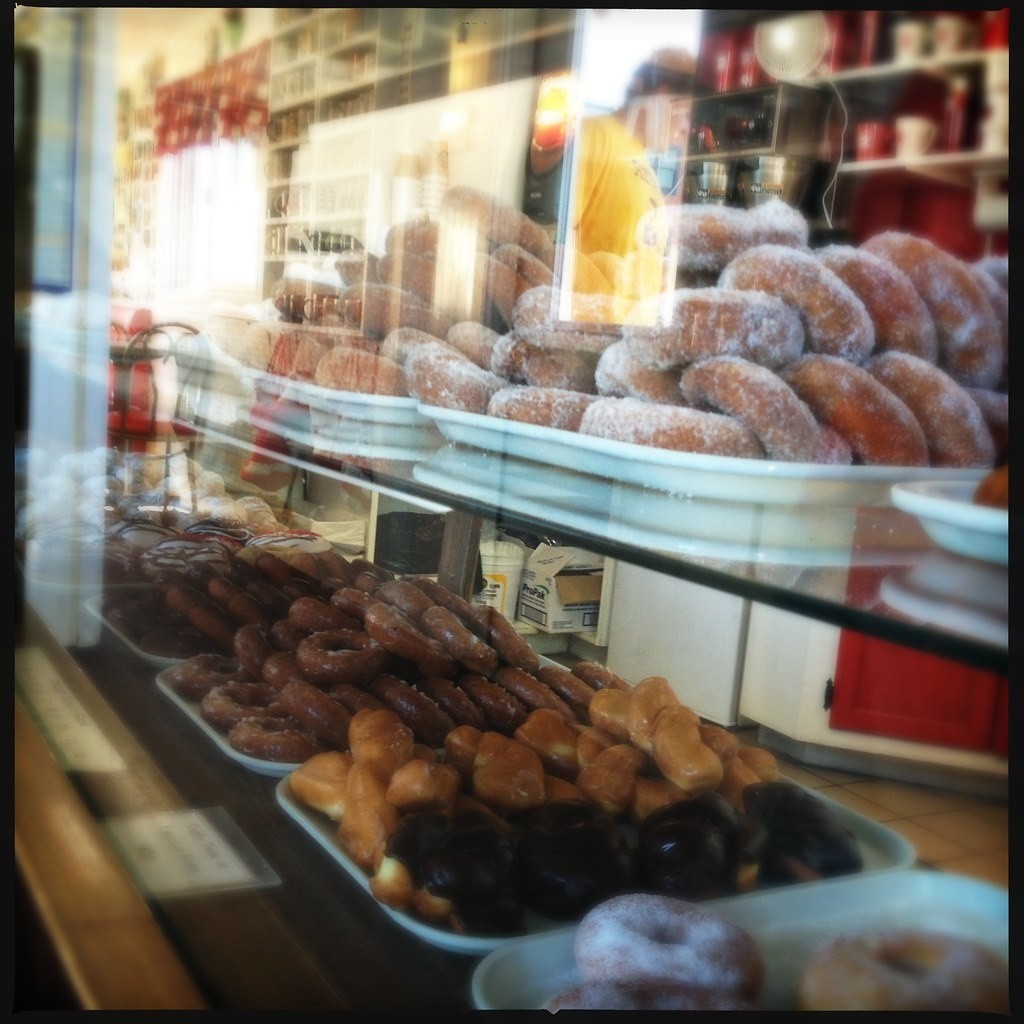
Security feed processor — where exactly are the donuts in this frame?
[14,435,1009,1011]
[217,180,1007,515]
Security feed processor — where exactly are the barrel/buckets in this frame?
[681,154,816,219]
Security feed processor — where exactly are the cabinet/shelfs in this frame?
[108,11,1009,795]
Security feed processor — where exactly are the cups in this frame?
[896,116,936,161]
[469,541,524,626]
[688,102,777,153]
[266,18,376,331]
[932,16,966,59]
[891,22,930,60]
[854,121,894,162]
